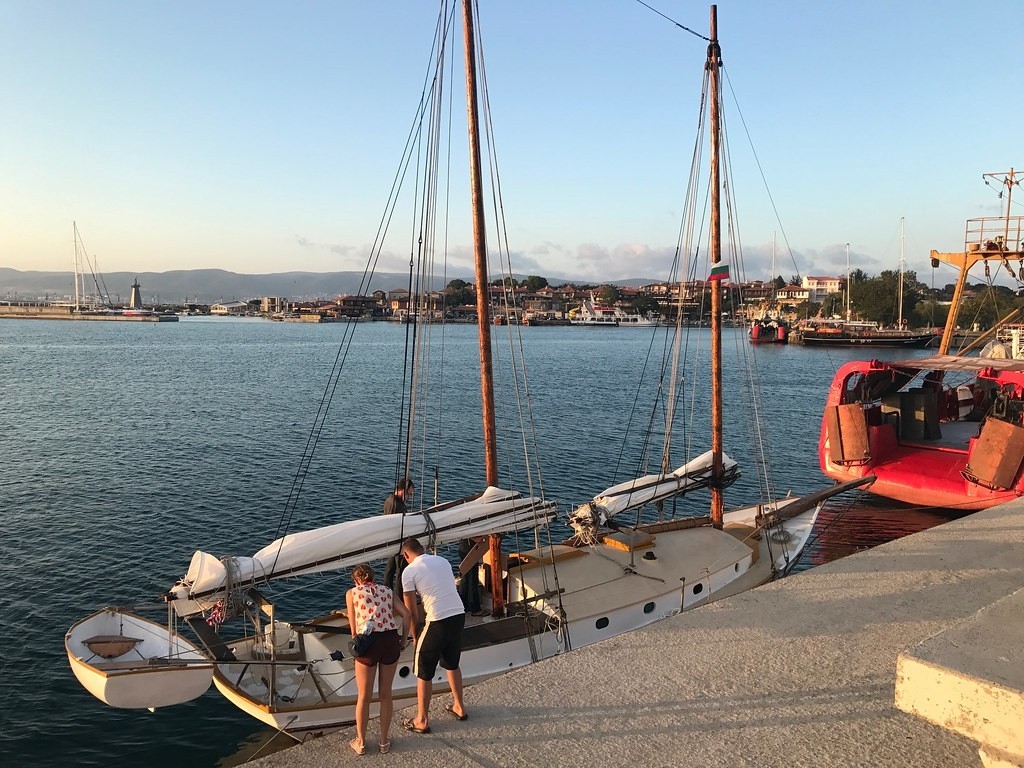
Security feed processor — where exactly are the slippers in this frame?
[402,718,430,733]
[471,610,492,616]
[447,704,469,720]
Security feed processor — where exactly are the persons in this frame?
[705,261,729,282]
[346,563,413,754]
[459,539,490,617]
[399,539,469,733]
[384,478,415,603]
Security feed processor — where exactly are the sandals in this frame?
[378,738,391,753]
[351,738,366,755]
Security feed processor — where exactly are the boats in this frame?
[802,318,938,349]
[569,314,658,327]
[819,354,1024,509]
[63,604,214,713]
[749,318,787,344]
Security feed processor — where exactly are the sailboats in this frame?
[162,2,881,742]
